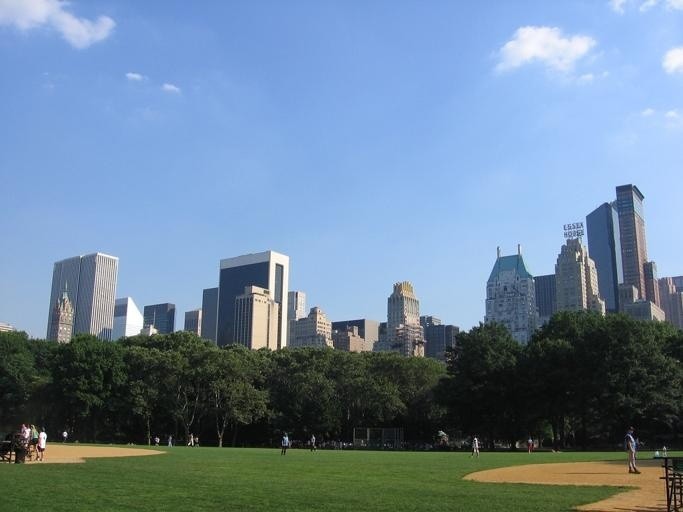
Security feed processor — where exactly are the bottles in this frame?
[663,446,666,457]
[655,450,659,457]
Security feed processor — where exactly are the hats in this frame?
[628,426,635,430]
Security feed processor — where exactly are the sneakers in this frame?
[629,470,641,474]
[36,458,43,461]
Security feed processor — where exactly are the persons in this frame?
[153,435,159,446]
[167,435,172,447]
[17,424,47,461]
[62,430,67,443]
[194,435,199,447]
[469,434,480,458]
[623,425,642,474]
[526,436,532,452]
[187,433,194,446]
[280,432,289,455]
[310,433,316,452]
